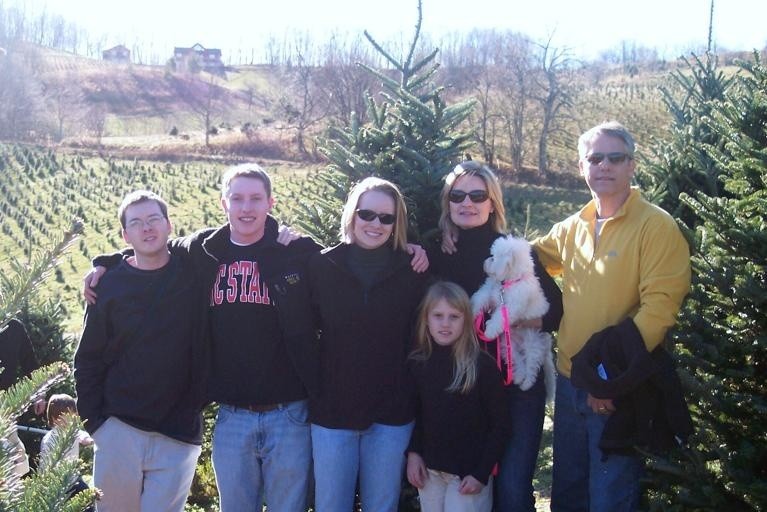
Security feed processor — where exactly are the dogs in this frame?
[471,234,556,413]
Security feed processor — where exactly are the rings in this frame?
[598,406,607,410]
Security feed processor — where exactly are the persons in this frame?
[72,190,299,512]
[435,118,693,511]
[301,174,436,511]
[83,163,433,512]
[14,391,96,512]
[422,160,564,511]
[0,294,48,418]
[403,277,516,512]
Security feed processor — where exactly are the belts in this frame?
[233,402,285,413]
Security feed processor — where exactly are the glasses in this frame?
[354,208,397,225]
[126,215,166,231]
[584,151,631,166]
[447,190,489,204]
